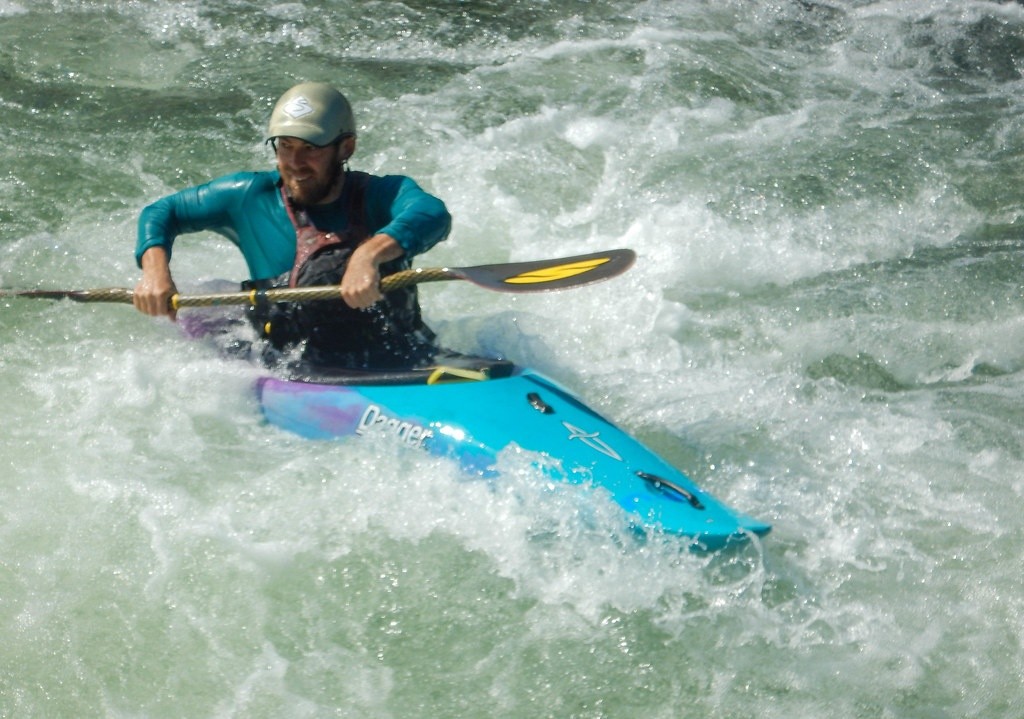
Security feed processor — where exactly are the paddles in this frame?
[0,246,637,305]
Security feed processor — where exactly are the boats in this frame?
[177,279,773,557]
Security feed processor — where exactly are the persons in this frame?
[136,83,453,382]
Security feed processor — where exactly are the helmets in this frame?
[265,82,357,147]
